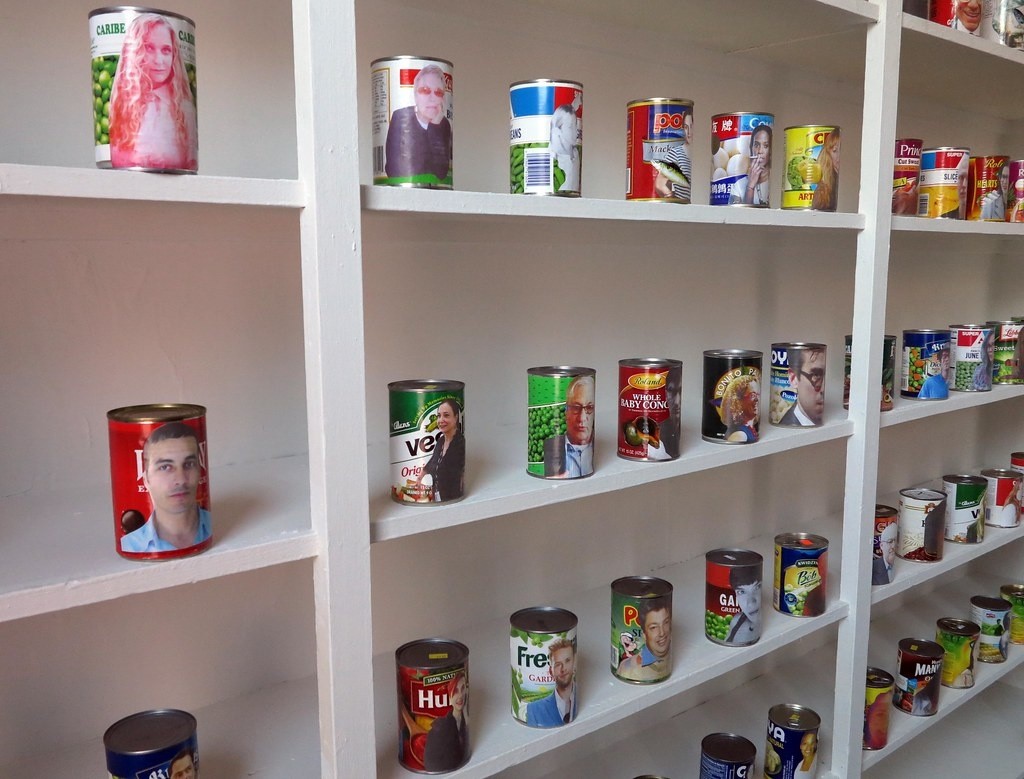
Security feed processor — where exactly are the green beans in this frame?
[705,610,734,641]
[91,55,121,145]
[527,403,567,462]
[184,63,197,99]
[955,361,981,390]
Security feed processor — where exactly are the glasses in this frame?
[570,403,595,414]
[997,174,1010,182]
[797,371,825,389]
[417,86,445,97]
[882,536,898,546]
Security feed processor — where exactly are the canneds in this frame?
[387,342,826,507]
[861,584,1024,750]
[395,576,673,774]
[103,708,201,779]
[369,53,842,215]
[927,0,1024,51]
[890,137,1024,223]
[87,6,200,175]
[632,704,821,779]
[844,319,1024,585]
[706,532,830,647]
[107,403,213,561]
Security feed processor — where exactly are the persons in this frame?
[424,671,472,773]
[617,597,673,680]
[120,422,214,552]
[647,367,682,461]
[527,639,580,726]
[722,375,761,444]
[385,65,453,180]
[801,552,828,615]
[778,347,826,426]
[810,127,842,212]
[722,562,763,645]
[168,745,196,779]
[545,375,595,478]
[733,734,819,779]
[859,126,1024,751]
[413,398,466,503]
[549,104,580,191]
[109,13,198,169]
[726,126,770,208]
[952,0,1024,51]
[655,113,694,201]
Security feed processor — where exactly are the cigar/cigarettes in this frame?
[749,156,759,158]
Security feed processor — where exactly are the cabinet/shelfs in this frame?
[0,0,1024,779]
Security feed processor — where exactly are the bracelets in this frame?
[747,185,754,191]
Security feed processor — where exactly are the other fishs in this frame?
[650,158,691,189]
